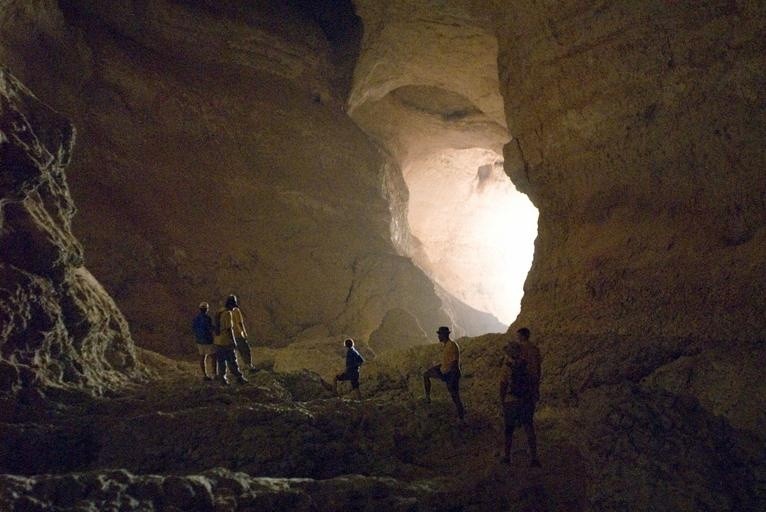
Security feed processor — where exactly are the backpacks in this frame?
[505,359,531,396]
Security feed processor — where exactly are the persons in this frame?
[423,326,465,423]
[194,293,260,385]
[500,327,542,468]
[332,338,364,401]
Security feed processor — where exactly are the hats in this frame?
[437,326,451,335]
[199,302,209,309]
[227,295,240,307]
